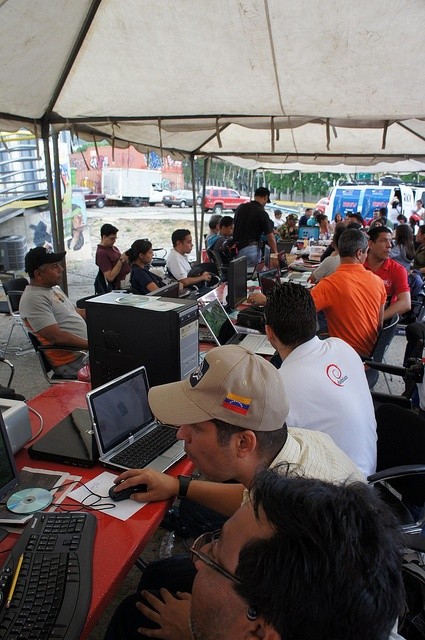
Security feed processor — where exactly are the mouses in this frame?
[108,481,150,502]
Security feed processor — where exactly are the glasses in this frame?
[189,528,243,587]
[142,238,149,244]
[107,236,118,240]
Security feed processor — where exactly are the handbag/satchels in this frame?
[196,262,219,287]
[236,240,248,249]
[408,271,423,297]
[166,265,205,290]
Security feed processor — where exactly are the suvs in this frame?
[72,187,106,209]
[197,186,251,214]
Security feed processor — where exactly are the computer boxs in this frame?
[86,292,199,389]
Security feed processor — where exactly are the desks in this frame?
[0,381,196,639]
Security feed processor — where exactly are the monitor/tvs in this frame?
[145,281,179,298]
[263,244,270,271]
[0,408,21,503]
[224,256,248,311]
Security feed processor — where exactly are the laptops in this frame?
[85,367,187,473]
[258,269,283,285]
[28,406,100,468]
[278,250,313,282]
[296,227,319,243]
[199,298,276,357]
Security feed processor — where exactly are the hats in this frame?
[287,214,301,223]
[25,246,67,272]
[255,186,272,203]
[147,343,290,432]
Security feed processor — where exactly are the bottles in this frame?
[309,236,314,247]
[304,238,308,248]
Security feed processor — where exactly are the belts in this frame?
[249,241,258,245]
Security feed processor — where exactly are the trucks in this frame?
[325,174,424,233]
[101,167,164,205]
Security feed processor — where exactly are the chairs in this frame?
[1,278,30,359]
[0,356,25,401]
[355,290,425,640]
[206,249,222,279]
[27,332,89,384]
[151,248,167,272]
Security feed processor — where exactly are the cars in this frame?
[162,189,194,208]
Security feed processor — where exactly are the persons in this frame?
[219,215,238,264]
[165,228,212,289]
[361,226,412,325]
[204,214,222,249]
[273,206,332,242]
[396,214,413,231]
[261,282,379,479]
[102,344,373,640]
[390,222,415,270]
[186,460,408,640]
[333,207,394,231]
[412,199,425,225]
[411,224,424,273]
[124,238,167,296]
[232,187,278,269]
[94,223,165,291]
[17,246,89,380]
[245,228,388,362]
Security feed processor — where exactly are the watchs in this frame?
[176,474,193,500]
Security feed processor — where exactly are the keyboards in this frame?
[1,511,96,640]
[198,329,215,342]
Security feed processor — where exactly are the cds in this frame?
[119,295,149,306]
[7,488,53,513]
